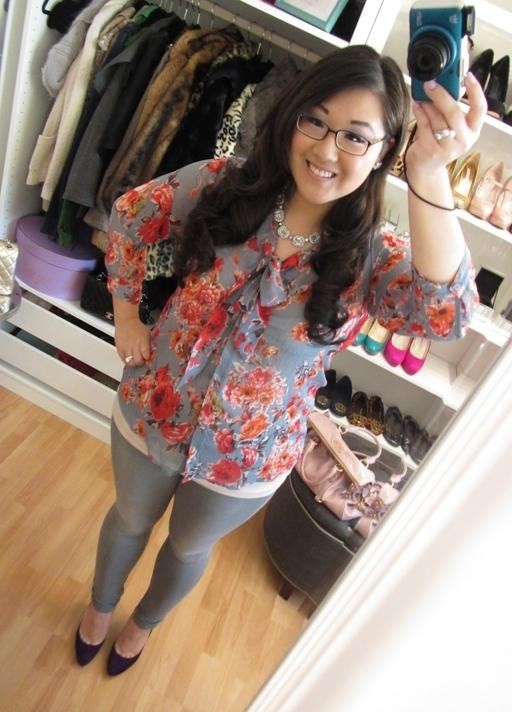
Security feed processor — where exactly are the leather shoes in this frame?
[74,623,109,666]
[107,627,155,676]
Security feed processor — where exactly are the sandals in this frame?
[352,314,432,375]
[412,428,440,463]
[314,369,418,449]
[462,47,511,126]
[389,118,512,235]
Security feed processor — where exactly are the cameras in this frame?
[407,1,476,105]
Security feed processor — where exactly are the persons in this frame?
[72,42,490,676]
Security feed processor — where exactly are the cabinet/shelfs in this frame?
[0,0,511,471]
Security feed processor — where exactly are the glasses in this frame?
[295,111,386,157]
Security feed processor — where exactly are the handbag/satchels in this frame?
[0,239,19,296]
[79,270,151,327]
[352,459,414,538]
[293,408,383,522]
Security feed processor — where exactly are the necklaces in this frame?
[273,178,327,248]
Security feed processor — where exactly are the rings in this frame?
[433,128,452,141]
[125,356,134,363]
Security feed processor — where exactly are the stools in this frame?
[261,429,407,622]
